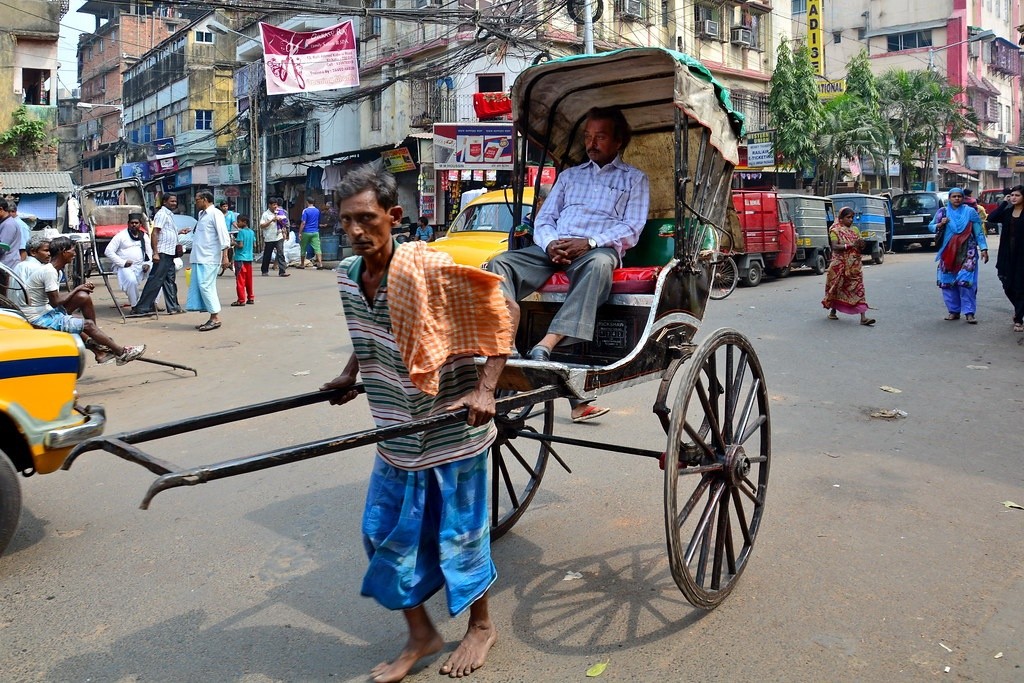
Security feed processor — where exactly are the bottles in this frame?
[186,268,192,285]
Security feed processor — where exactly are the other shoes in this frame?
[296,265,305,269]
[130,304,187,315]
[965,314,977,324]
[944,314,960,321]
[279,273,290,277]
[262,273,269,276]
[317,266,323,270]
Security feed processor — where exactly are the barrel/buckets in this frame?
[320,237,340,260]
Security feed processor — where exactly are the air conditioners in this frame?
[415,0,442,11]
[614,0,642,18]
[695,18,719,38]
[731,28,752,46]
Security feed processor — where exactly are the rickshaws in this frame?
[75,175,163,324]
[59,45,774,610]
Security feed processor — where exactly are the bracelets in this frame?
[845,244,851,249]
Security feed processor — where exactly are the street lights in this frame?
[76,101,128,164]
[930,29,999,198]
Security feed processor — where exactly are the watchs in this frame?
[587,236,597,249]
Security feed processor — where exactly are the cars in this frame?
[427,187,536,271]
[0,303,107,557]
[974,188,1005,214]
[890,190,950,251]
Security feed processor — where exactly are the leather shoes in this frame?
[530,349,549,362]
[509,347,522,359]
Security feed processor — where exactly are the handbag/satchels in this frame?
[934,207,946,249]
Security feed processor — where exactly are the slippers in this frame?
[511,408,524,413]
[1014,322,1023,332]
[98,352,114,364]
[861,318,876,325]
[828,314,838,319]
[196,320,221,331]
[572,406,610,422]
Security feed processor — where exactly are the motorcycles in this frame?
[715,188,895,285]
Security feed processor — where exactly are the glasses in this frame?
[195,197,203,201]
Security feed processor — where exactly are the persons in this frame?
[521,183,611,422]
[319,159,520,683]
[0,190,337,366]
[414,215,434,243]
[821,207,876,326]
[485,108,649,363]
[928,185,1024,331]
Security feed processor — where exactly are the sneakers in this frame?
[85,335,106,349]
[115,344,146,366]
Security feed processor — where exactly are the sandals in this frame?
[246,299,254,304]
[231,301,246,306]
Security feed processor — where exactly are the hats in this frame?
[128,213,143,223]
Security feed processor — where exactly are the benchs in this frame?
[89,204,149,243]
[534,217,717,293]
